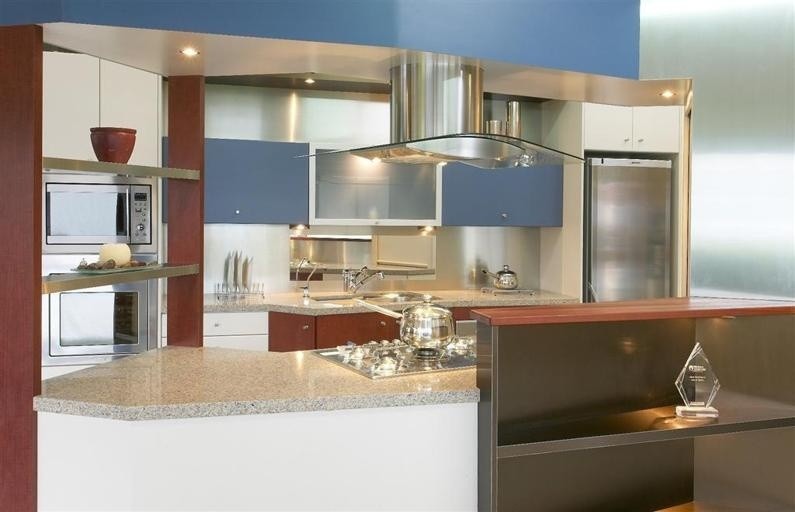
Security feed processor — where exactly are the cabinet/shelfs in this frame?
[162,137,309,224]
[442,153,563,226]
[539,99,684,154]
[469,299,795,511]
[159,306,469,352]
[1,26,204,511]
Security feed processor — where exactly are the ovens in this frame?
[41,253,157,367]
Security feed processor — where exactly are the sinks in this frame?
[312,291,442,302]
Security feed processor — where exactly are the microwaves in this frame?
[44,182,154,246]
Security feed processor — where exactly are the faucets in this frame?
[296,257,306,279]
[354,271,385,295]
[307,265,320,288]
[354,266,367,282]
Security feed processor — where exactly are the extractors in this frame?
[291,61,588,172]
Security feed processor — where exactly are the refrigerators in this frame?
[583,156,674,307]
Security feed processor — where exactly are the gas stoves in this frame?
[311,334,478,380]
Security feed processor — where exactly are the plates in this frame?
[226,252,249,295]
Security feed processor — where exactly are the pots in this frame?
[352,294,457,351]
[482,264,519,290]
